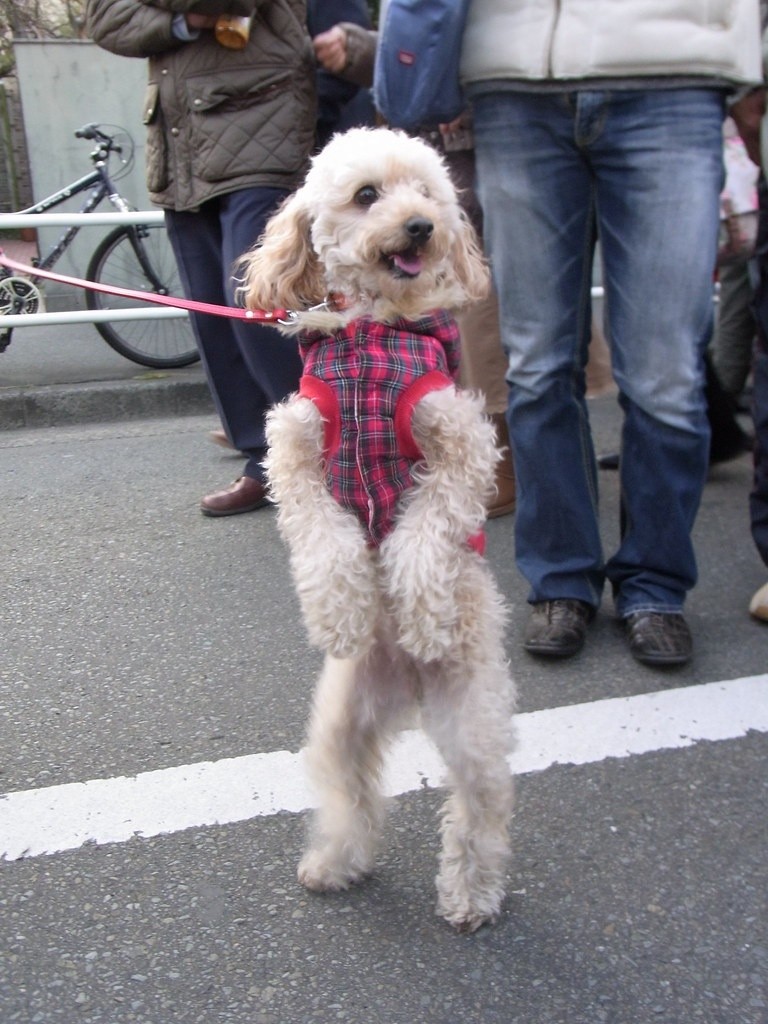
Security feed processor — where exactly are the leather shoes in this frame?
[200,474,271,517]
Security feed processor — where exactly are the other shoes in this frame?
[597,451,620,469]
[748,581,768,621]
[207,429,234,450]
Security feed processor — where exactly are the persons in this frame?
[87,0,768,666]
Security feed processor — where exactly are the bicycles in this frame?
[1,121,209,373]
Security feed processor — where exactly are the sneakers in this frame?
[629,612,694,667]
[522,598,592,659]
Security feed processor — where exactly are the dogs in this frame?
[224,125,522,933]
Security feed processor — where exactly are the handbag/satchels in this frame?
[373,1,470,127]
